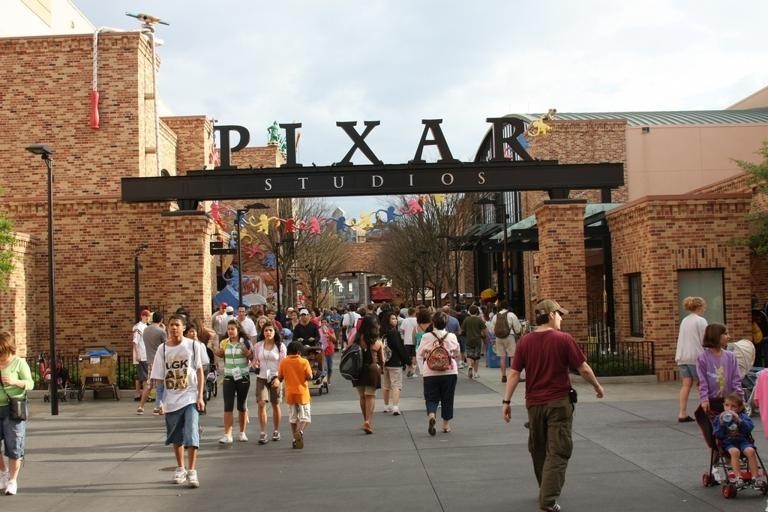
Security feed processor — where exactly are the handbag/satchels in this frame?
[8,398,26,422]
[340,344,362,381]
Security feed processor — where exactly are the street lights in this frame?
[238,201,272,309]
[409,259,426,306]
[24,143,66,416]
[419,250,438,308]
[471,197,510,310]
[435,233,462,304]
[275,237,298,314]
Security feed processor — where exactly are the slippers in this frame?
[679,416,695,421]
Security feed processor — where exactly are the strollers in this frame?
[202,347,218,400]
[724,338,761,416]
[692,397,768,500]
[297,339,330,395]
[36,348,71,403]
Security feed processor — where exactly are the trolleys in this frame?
[76,345,122,401]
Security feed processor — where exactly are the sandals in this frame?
[152,408,160,415]
[136,407,144,414]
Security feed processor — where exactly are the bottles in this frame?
[721,409,733,424]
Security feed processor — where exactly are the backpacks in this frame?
[426,331,452,370]
[495,310,512,338]
[381,330,396,363]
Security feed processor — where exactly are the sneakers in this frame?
[362,421,372,433]
[135,394,156,402]
[541,503,560,511]
[502,376,507,382]
[173,468,199,488]
[384,404,401,415]
[519,378,526,382]
[292,429,303,449]
[0,468,17,494]
[428,415,436,435]
[219,431,281,443]
[440,425,450,433]
[458,360,480,378]
[712,467,763,491]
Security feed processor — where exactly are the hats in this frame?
[220,303,234,312]
[141,310,150,317]
[534,298,569,317]
[300,309,308,315]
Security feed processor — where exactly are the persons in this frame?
[502,299,603,511]
[696,323,743,485]
[714,394,760,489]
[1,331,34,494]
[672,297,709,422]
[132,299,527,489]
